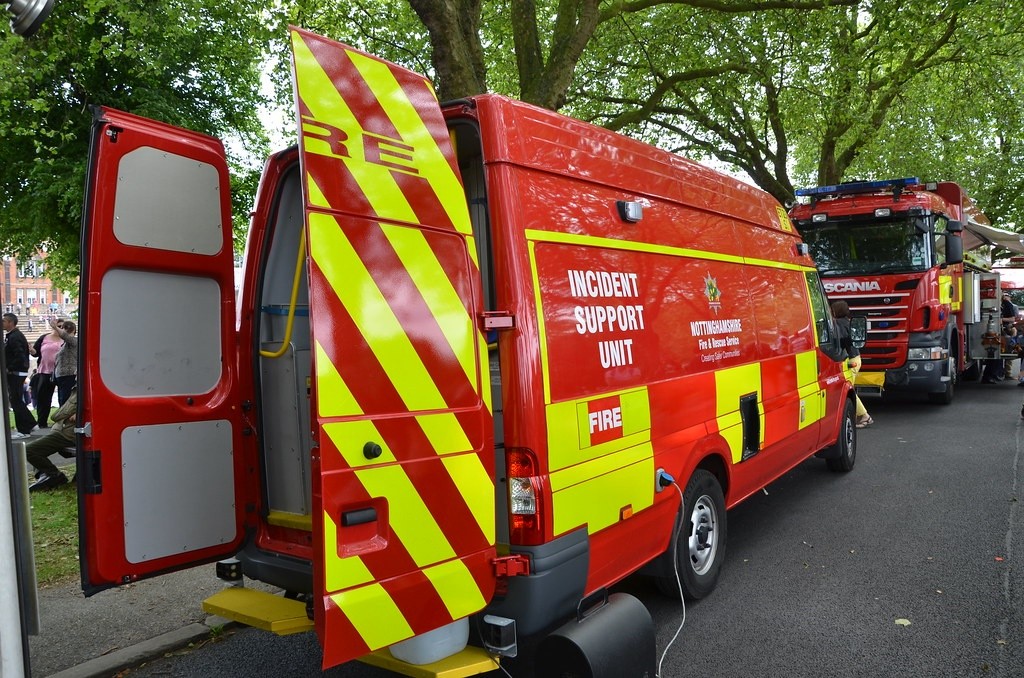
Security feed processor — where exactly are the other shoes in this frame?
[29,468,69,491]
[11,424,40,440]
[856,416,875,427]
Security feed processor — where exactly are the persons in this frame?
[27,319,67,429]
[5,295,78,334]
[46,314,78,411]
[982,293,1019,384]
[2,312,39,439]
[25,369,77,492]
[832,299,875,429]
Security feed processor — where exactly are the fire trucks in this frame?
[77,27,866,676]
[787,177,1023,405]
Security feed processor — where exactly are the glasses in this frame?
[57,325,63,329]
